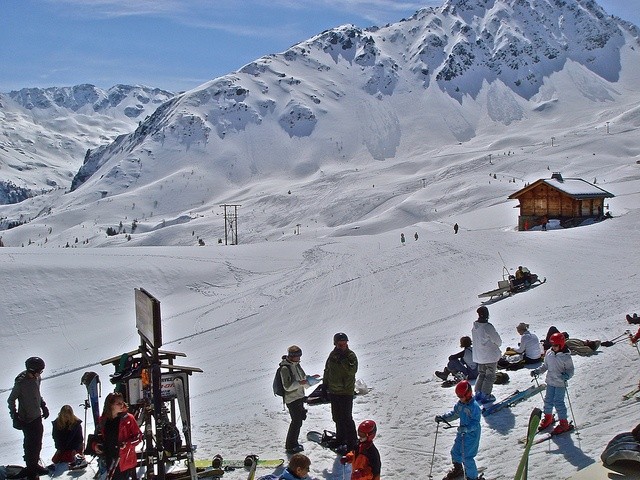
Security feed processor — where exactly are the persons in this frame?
[52,405,86,465]
[322,332,358,454]
[470,306,502,402]
[280,345,320,454]
[435,337,479,379]
[530,333,573,434]
[92,392,143,479]
[276,455,318,480]
[158,417,182,454]
[507,323,542,364]
[8,357,50,479]
[544,326,569,350]
[435,381,485,479]
[516,266,525,280]
[340,420,381,479]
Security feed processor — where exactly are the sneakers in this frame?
[26,465,39,480]
[286,445,303,453]
[475,391,482,400]
[35,458,49,475]
[540,414,553,428]
[554,420,569,434]
[447,462,463,477]
[482,394,496,404]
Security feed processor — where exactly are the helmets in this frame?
[334,333,349,345]
[549,332,566,348]
[26,356,45,372]
[358,420,376,442]
[455,381,472,399]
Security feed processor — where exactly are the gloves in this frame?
[457,427,467,433]
[531,370,535,377]
[435,417,443,422]
[353,468,364,477]
[601,424,640,465]
[561,372,569,380]
[41,402,49,419]
[13,413,23,430]
[340,456,348,464]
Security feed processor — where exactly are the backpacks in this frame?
[273,364,294,398]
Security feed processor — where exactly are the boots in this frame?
[435,367,451,380]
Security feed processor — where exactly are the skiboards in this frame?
[518,414,574,448]
[624,382,640,400]
[141,363,166,480]
[442,473,486,480]
[174,376,199,480]
[482,384,547,417]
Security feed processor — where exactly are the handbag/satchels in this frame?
[493,372,509,384]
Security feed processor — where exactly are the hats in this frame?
[287,346,302,357]
[477,307,489,318]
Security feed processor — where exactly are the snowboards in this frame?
[184,459,285,468]
[306,431,341,452]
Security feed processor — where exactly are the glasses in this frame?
[550,344,558,347]
[357,432,368,438]
[457,384,471,398]
